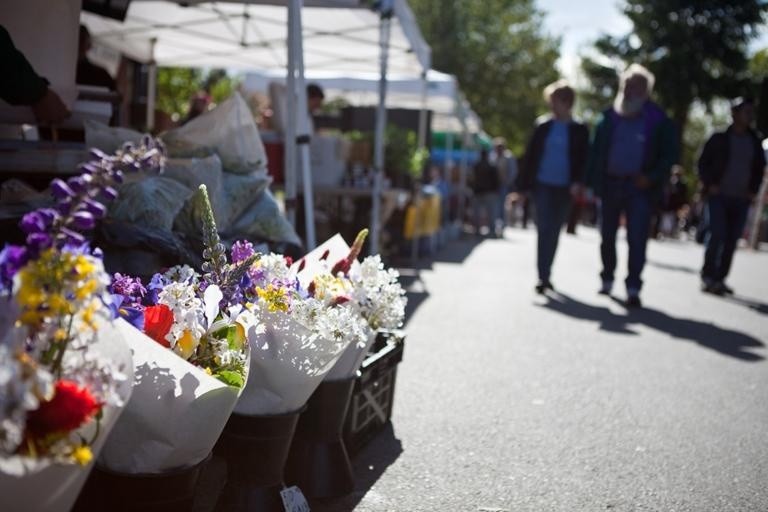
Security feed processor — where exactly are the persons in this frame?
[1,21,69,249]
[39,22,122,146]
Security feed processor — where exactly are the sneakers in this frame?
[626,280,647,307]
[598,272,616,292]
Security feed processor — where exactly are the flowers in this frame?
[0,124,408,465]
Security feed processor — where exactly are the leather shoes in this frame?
[703,280,734,297]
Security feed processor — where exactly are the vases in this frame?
[70,375,357,512]
